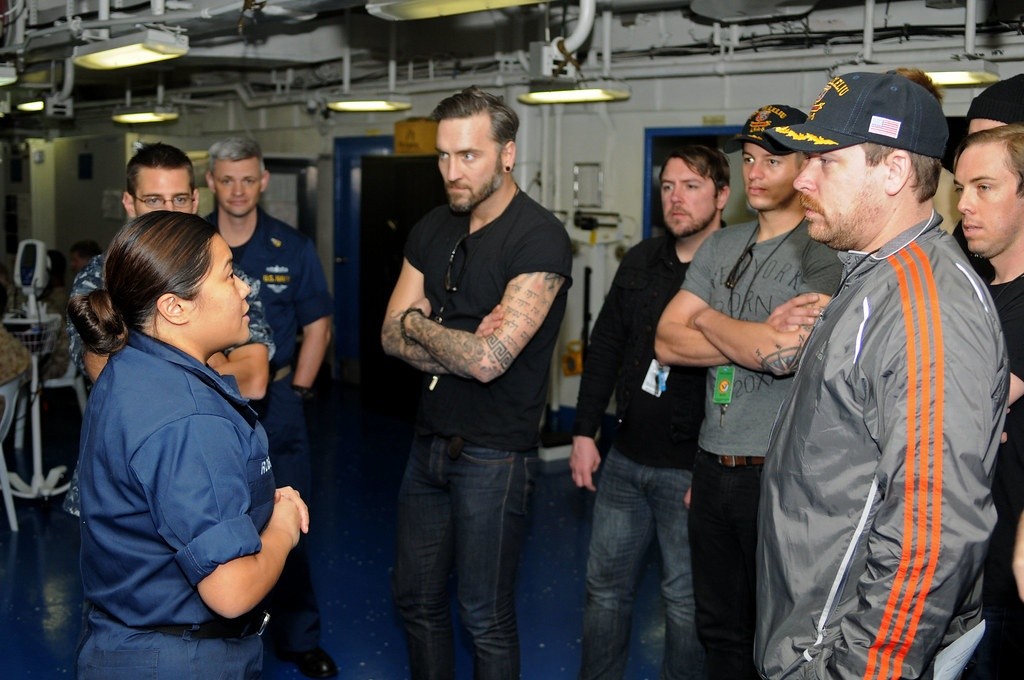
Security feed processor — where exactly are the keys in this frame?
[720,399,728,429]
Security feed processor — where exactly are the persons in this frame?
[67,211,310,680]
[882,67,1024,680]
[0,237,114,459]
[569,143,730,680]
[204,138,338,680]
[381,85,573,680]
[655,106,845,680]
[67,143,276,434]
[755,72,1010,680]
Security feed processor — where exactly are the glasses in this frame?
[445,231,471,292]
[134,196,195,209]
[724,241,757,290]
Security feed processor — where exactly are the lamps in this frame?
[365,1,547,22]
[326,92,412,111]
[71,28,189,70]
[111,103,178,123]
[517,76,632,105]
[830,59,1002,87]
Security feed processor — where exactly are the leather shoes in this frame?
[284,647,338,680]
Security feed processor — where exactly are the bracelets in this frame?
[400,308,427,345]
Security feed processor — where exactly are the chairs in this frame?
[0,337,91,532]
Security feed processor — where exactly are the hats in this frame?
[724,104,810,156]
[762,70,949,159]
[966,71,1024,125]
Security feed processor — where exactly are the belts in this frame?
[718,454,766,467]
[152,610,271,636]
